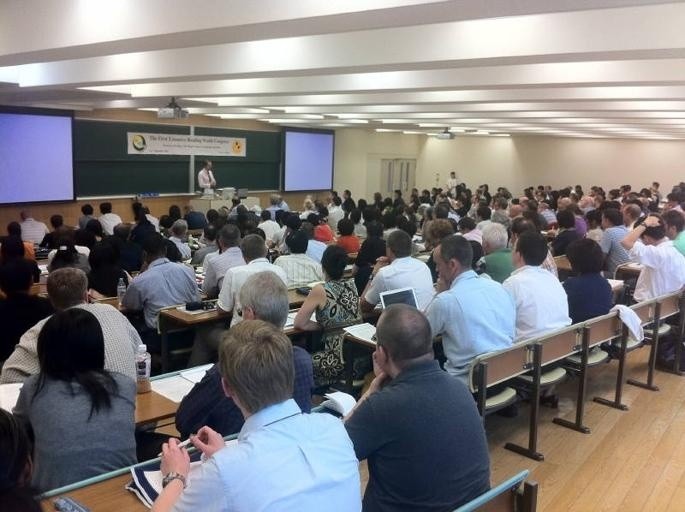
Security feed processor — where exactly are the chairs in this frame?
[0,223,685,512]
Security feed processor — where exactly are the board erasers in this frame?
[139,191,159,197]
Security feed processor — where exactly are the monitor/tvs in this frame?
[224,188,234,199]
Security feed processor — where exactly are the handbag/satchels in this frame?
[656,334,685,370]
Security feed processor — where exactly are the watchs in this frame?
[368,275,374,280]
[641,222,648,229]
[162,472,187,489]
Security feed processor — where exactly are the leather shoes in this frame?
[541,394,558,407]
[498,407,517,417]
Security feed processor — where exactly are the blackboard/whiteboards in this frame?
[73,117,284,201]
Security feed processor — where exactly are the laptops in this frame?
[235,189,248,199]
[380,287,420,311]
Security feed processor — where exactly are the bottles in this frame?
[141,192,160,197]
[188,234,193,246]
[134,344,152,393]
[118,277,127,305]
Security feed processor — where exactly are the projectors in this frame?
[158,108,188,119]
[439,133,454,139]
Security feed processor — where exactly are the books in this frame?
[320,391,357,417]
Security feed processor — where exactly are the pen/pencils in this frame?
[157,439,191,457]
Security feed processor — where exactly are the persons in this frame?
[198,159,216,194]
[0,173,685,511]
